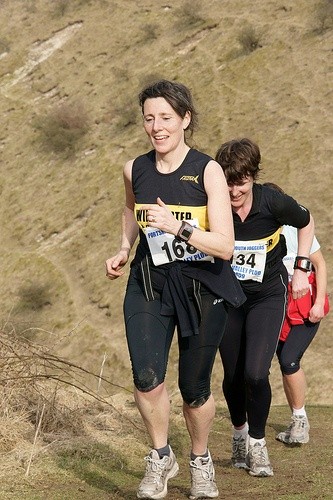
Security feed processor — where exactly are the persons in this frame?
[262,182,329,445]
[215,137,315,476]
[104,79,235,500]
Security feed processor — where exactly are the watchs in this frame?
[293,256,312,272]
[176,221,193,241]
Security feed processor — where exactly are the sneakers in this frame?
[188,449,220,498]
[289,415,310,444]
[276,427,291,444]
[231,434,248,469]
[137,444,179,499]
[247,440,274,477]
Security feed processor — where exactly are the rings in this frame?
[152,216,156,221]
